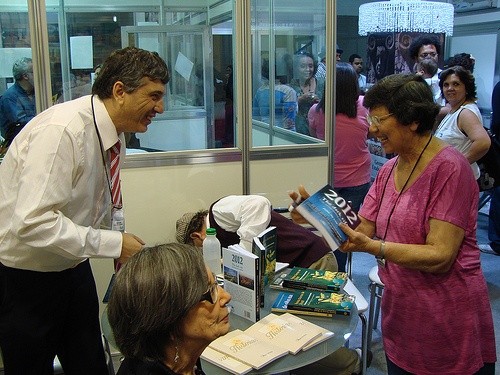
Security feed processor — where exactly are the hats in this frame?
[318,44,344,58]
[176,212,197,245]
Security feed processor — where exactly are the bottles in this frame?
[202,228,222,275]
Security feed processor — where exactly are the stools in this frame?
[368,265,385,329]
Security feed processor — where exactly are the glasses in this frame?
[353,62,363,65]
[366,112,395,125]
[199,275,224,304]
[417,52,439,59]
[297,50,306,55]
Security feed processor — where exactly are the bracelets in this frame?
[375,239,385,260]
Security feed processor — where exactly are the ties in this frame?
[109,141,123,214]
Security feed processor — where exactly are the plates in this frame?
[275,262,289,272]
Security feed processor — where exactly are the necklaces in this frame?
[297,80,311,94]
[434,100,467,136]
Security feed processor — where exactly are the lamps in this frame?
[358,0,454,37]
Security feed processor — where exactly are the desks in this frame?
[200,266,369,375]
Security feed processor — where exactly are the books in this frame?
[269,266,355,318]
[252,226,278,307]
[222,244,261,324]
[199,311,335,375]
[297,184,361,251]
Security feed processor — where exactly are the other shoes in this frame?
[477,244,500,255]
[351,347,373,375]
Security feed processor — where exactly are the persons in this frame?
[252,35,492,190]
[308,63,372,210]
[108,243,232,375]
[93,65,140,148]
[175,194,373,375]
[478,82,500,255]
[0,58,37,153]
[289,73,496,375]
[0,48,170,375]
[194,65,259,147]
